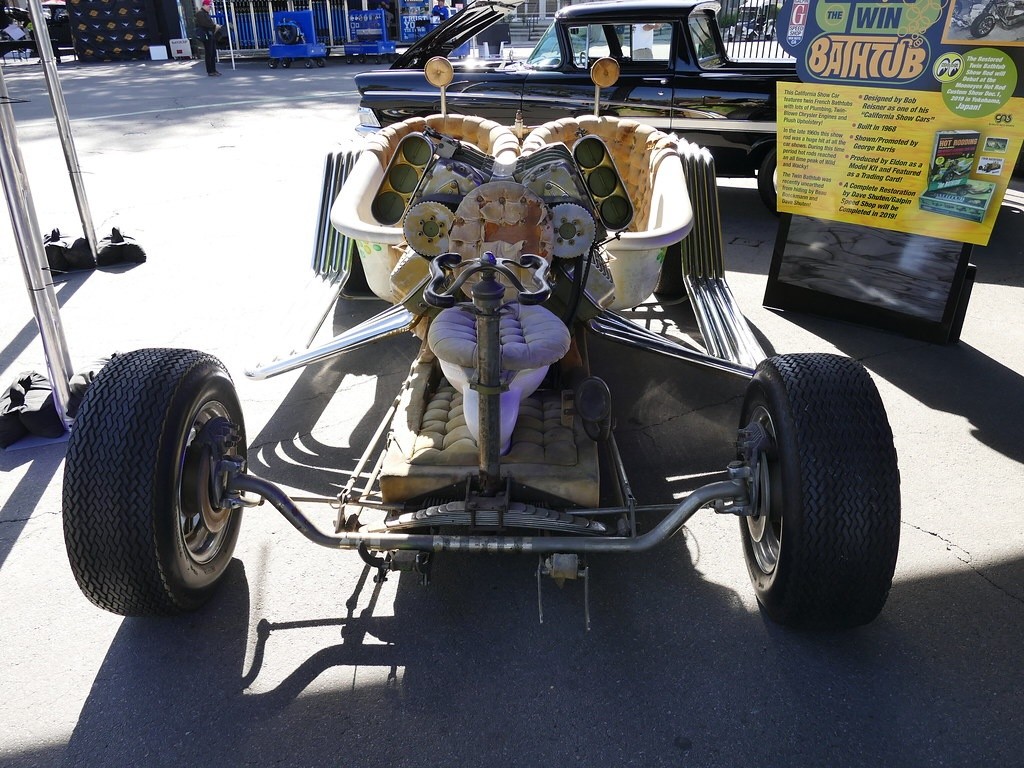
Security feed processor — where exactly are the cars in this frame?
[4,0,72,47]
[354,0,796,213]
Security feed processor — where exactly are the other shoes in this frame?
[208,71,221,76]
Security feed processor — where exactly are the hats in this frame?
[203,0,212,5]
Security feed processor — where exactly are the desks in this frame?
[0,39,61,65]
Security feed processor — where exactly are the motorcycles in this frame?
[941,158,959,182]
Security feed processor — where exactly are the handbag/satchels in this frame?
[192,28,212,41]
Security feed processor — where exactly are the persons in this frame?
[748,13,765,40]
[633,23,664,60]
[614,25,625,47]
[196,0,222,77]
[423,0,449,21]
